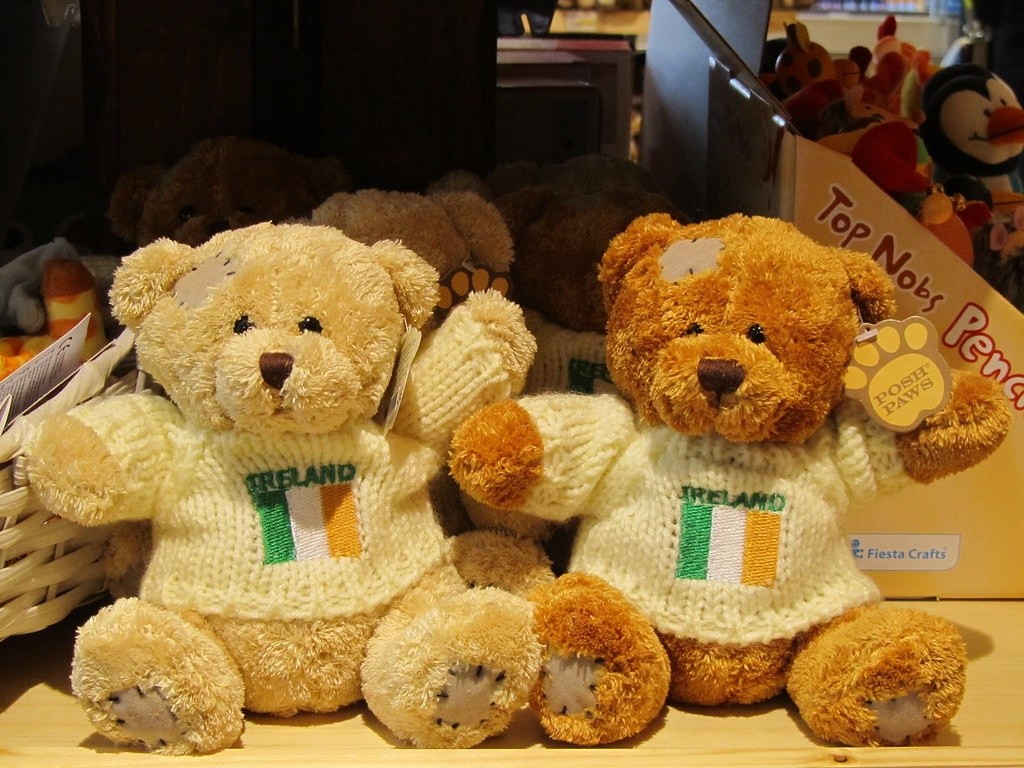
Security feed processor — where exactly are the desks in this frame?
[0,596,1024,768]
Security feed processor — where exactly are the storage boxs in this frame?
[0,256,164,643]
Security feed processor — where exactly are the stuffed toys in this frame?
[21,217,537,757]
[761,14,1023,310]
[447,211,1012,749]
[1,256,108,384]
[105,135,347,247]
[288,186,608,553]
[426,155,694,334]
